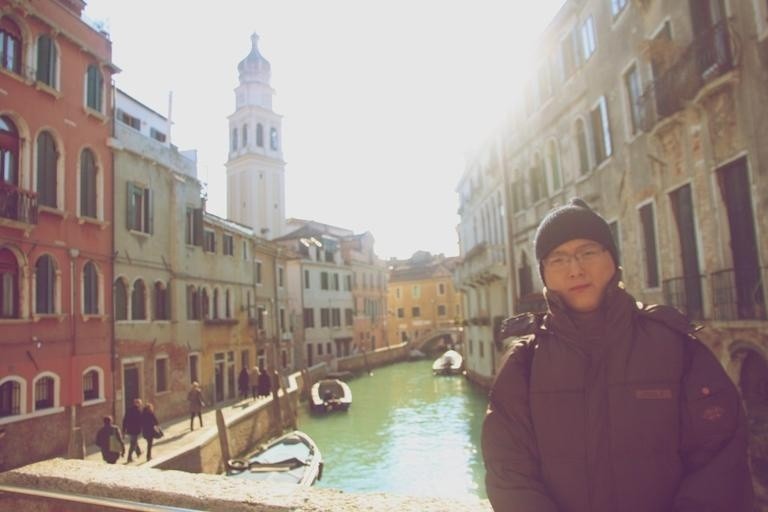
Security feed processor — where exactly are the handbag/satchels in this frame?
[152,429,162,439]
[109,435,121,454]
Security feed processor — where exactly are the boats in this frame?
[219,429,325,486]
[430,348,465,376]
[307,376,352,413]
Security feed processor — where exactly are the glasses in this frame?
[542,246,604,268]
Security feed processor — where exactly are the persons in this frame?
[482,198,754,512]
[95,416,124,465]
[143,402,158,462]
[122,397,143,462]
[187,382,204,430]
[237,366,271,400]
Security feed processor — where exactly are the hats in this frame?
[534,198,616,259]
